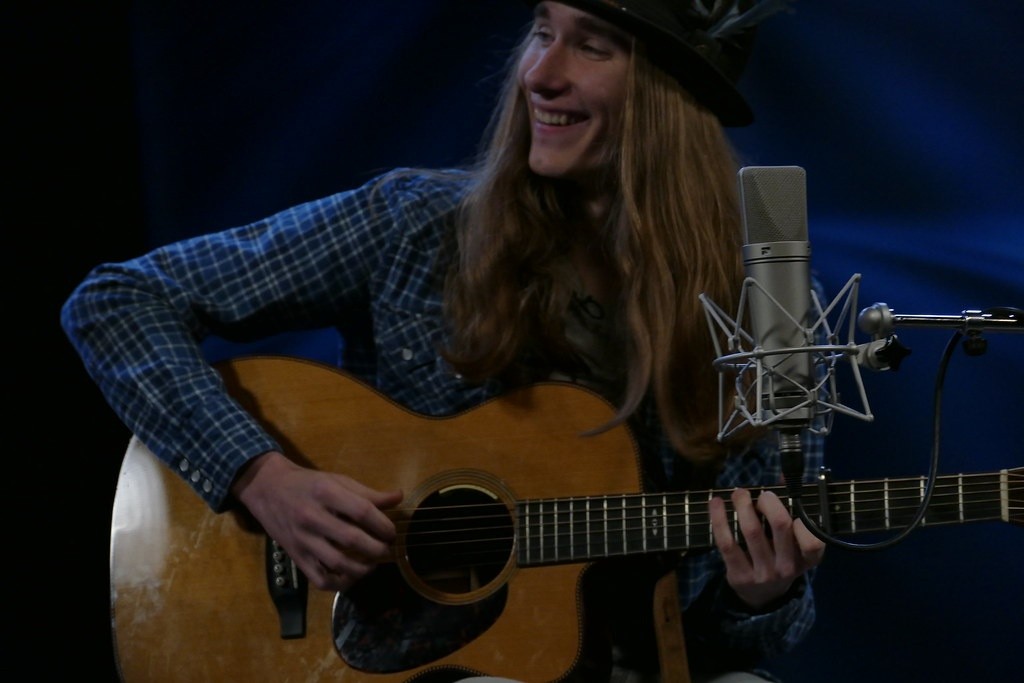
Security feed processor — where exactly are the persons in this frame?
[61,0,838,682]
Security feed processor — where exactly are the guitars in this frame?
[106,355,1024,683]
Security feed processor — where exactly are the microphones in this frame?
[733,163,814,423]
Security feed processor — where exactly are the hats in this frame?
[554,0,759,130]
